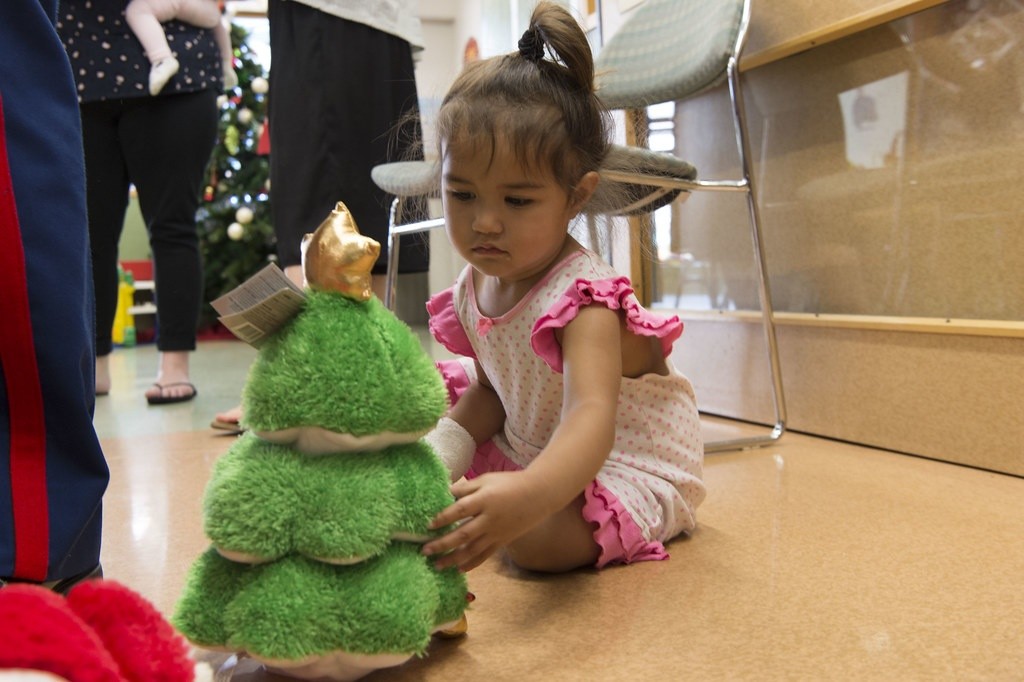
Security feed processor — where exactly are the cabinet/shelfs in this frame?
[623,1,1024,482]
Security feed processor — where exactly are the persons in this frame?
[1,0,108,597]
[49,21,222,404]
[210,1,424,428]
[123,0,240,98]
[373,1,706,577]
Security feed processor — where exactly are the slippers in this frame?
[210,411,246,432]
[145,380,198,405]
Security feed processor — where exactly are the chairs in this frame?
[368,0,787,457]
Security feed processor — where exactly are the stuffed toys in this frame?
[0,577,195,681]
[176,291,476,679]
